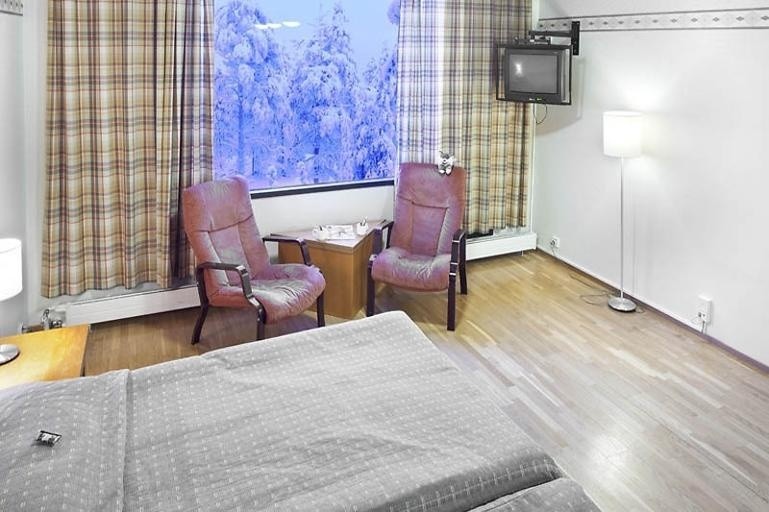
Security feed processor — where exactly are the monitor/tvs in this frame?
[504,43,571,106]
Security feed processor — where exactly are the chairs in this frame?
[180,176,326,341]
[366,162,466,331]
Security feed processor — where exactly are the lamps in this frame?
[0,237,22,365]
[602,109,650,316]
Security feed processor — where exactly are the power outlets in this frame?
[698,300,713,331]
[548,237,558,247]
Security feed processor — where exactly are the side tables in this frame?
[269,219,384,321]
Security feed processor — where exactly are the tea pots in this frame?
[312,228,335,241]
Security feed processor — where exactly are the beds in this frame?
[0,311,597,511]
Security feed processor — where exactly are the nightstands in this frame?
[1,322,92,382]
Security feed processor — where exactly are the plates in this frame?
[326,225,354,239]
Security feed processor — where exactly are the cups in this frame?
[357,223,368,235]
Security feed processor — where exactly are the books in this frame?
[322,224,354,240]
